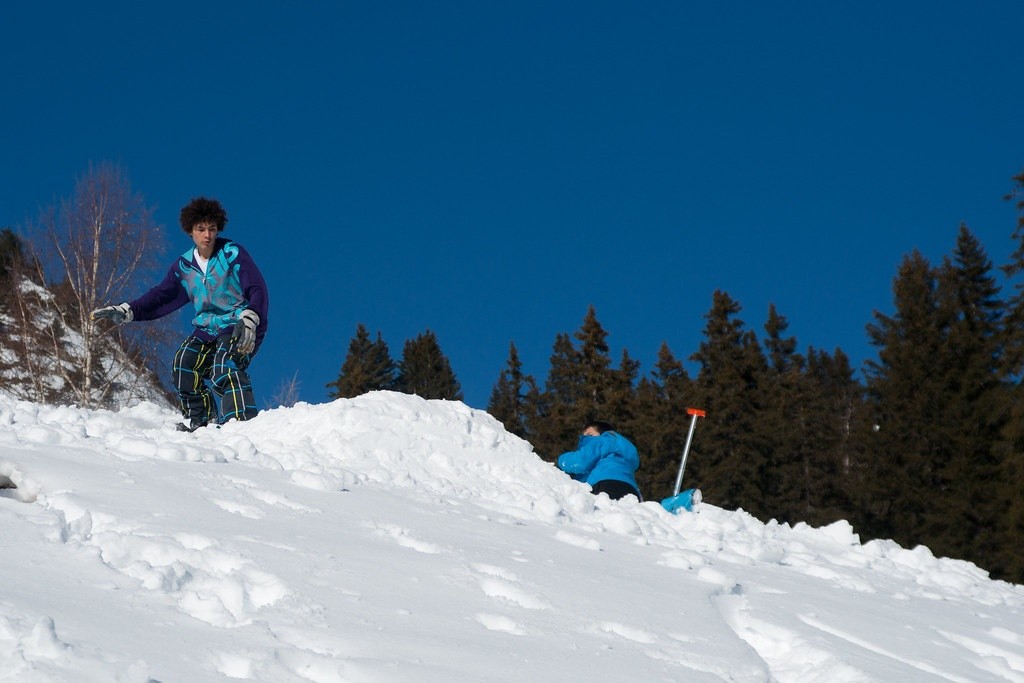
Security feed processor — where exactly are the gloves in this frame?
[90,303,133,324]
[232,309,261,354]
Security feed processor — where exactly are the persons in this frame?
[557,421,644,503]
[92,197,269,432]
